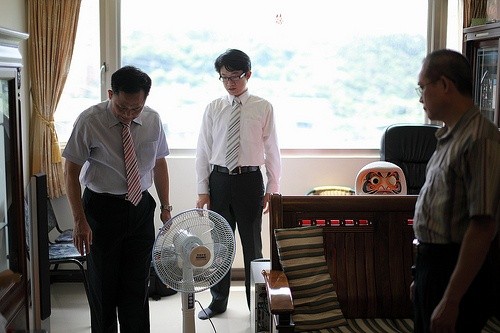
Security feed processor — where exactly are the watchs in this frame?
[160,205,173,211]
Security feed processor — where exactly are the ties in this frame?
[225,97,241,172]
[118,121,142,207]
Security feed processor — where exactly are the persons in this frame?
[61,67,171,333]
[410,50,500,333]
[195,50,281,318]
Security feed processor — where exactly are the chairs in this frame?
[306,186,355,195]
[380,125,442,196]
[49,195,89,305]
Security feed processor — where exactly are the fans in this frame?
[152,209,236,333]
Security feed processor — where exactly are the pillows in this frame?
[275,228,347,331]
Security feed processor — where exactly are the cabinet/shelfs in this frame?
[0,29,42,333]
[462,21,499,130]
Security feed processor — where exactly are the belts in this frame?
[214,165,260,175]
[86,186,147,201]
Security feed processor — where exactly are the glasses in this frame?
[414,78,442,97]
[219,72,246,82]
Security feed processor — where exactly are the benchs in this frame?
[262,195,500,333]
